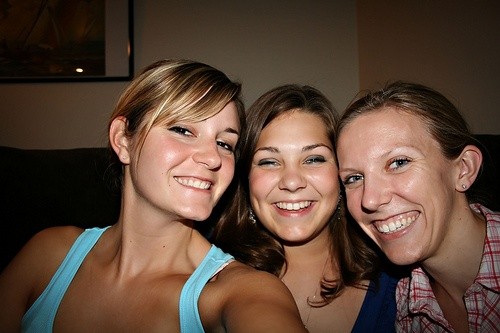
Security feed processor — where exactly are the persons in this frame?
[332,75,500,333]
[194,80,406,333]
[0,56,310,333]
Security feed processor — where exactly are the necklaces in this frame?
[275,242,334,330]
